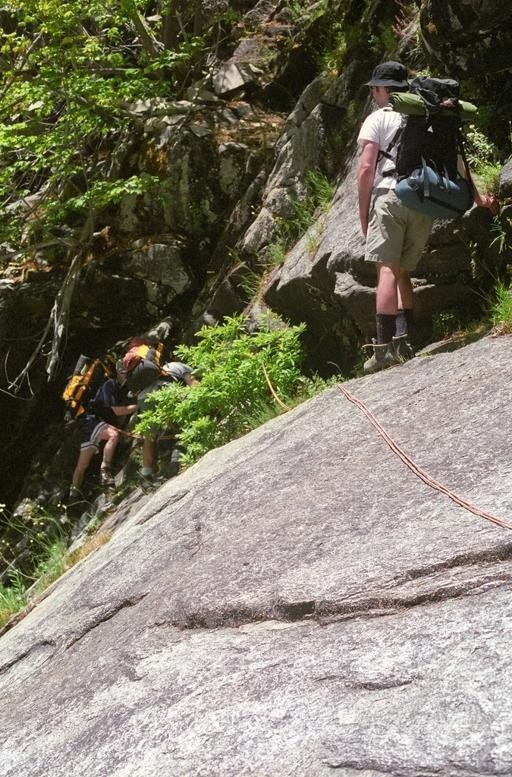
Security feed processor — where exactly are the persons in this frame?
[352,60,501,374]
[136,361,202,494]
[68,357,141,520]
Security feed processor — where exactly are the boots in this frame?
[363,333,412,371]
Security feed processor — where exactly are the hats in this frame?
[364,61,410,88]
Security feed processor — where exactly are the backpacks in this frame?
[115,338,174,397]
[390,75,475,220]
[61,350,117,421]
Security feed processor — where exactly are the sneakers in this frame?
[98,467,116,485]
[138,469,156,495]
[170,449,186,463]
